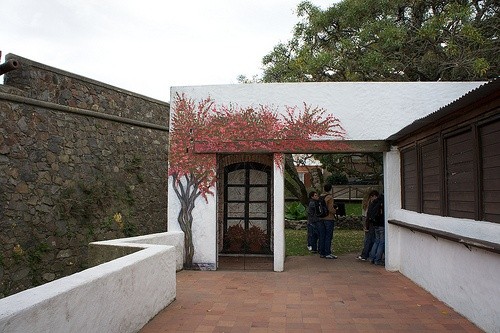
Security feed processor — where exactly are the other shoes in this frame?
[369,259,374,264]
[325,254,338,259]
[307,246,312,250]
[375,261,384,265]
[356,256,366,261]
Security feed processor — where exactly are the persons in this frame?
[307,191,319,251]
[356,187,385,265]
[319,183,338,259]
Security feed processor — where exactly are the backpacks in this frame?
[315,194,331,218]
